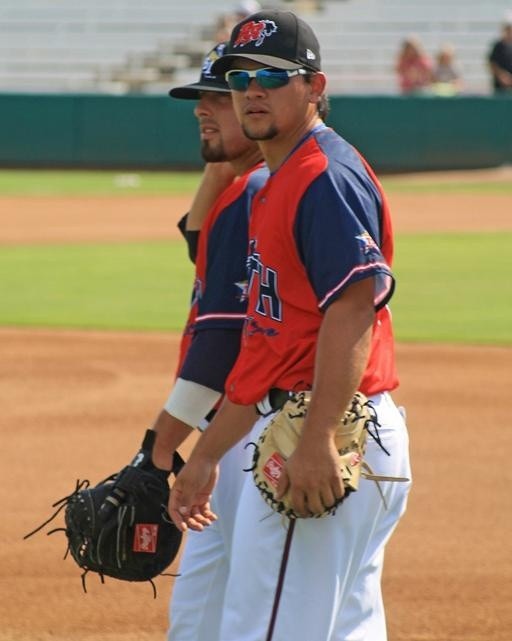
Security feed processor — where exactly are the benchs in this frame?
[0,0,512,112]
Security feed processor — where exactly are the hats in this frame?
[210,7,322,75]
[169,40,231,99]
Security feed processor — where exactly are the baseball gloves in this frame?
[65,429,186,582]
[252,390,370,519]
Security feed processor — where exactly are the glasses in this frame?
[225,63,314,90]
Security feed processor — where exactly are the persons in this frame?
[488,24,512,97]
[431,50,464,96]
[395,40,431,96]
[213,16,232,44]
[166,10,413,640]
[23,44,271,639]
[235,1,258,23]
